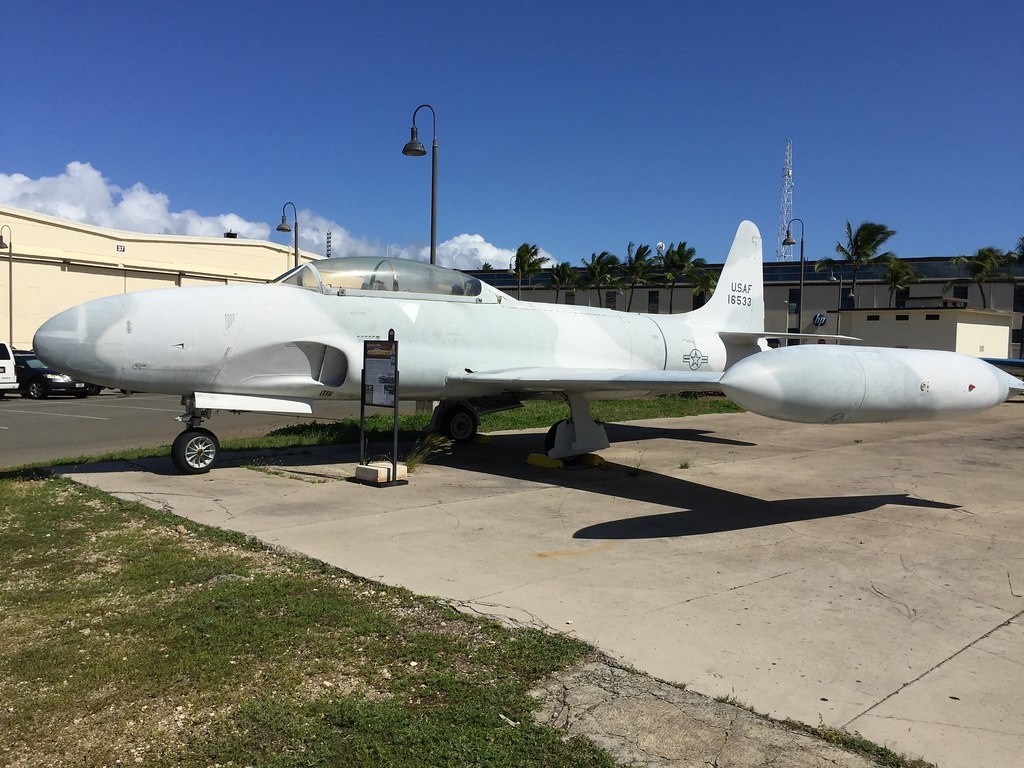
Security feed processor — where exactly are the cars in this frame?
[5,350,95,399]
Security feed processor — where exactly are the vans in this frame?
[0,342,20,399]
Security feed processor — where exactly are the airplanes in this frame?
[30,218,1024,476]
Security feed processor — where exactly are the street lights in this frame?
[277,201,300,270]
[0,224,14,350]
[401,104,438,266]
[828,262,843,345]
[507,255,521,300]
[781,218,805,346]
